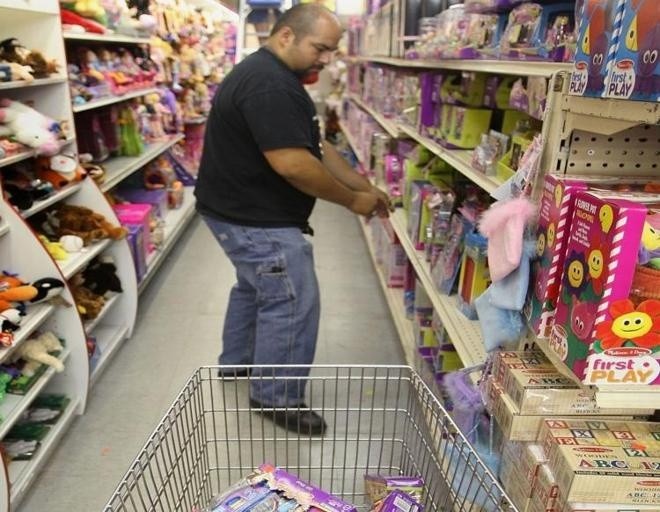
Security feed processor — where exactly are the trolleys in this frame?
[97,364,519,512]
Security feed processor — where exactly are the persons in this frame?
[196,2,394,435]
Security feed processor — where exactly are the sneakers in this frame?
[217,368,252,381]
[250,398,327,435]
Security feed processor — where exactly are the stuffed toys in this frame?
[1,0,240,454]
[0,0,240,455]
